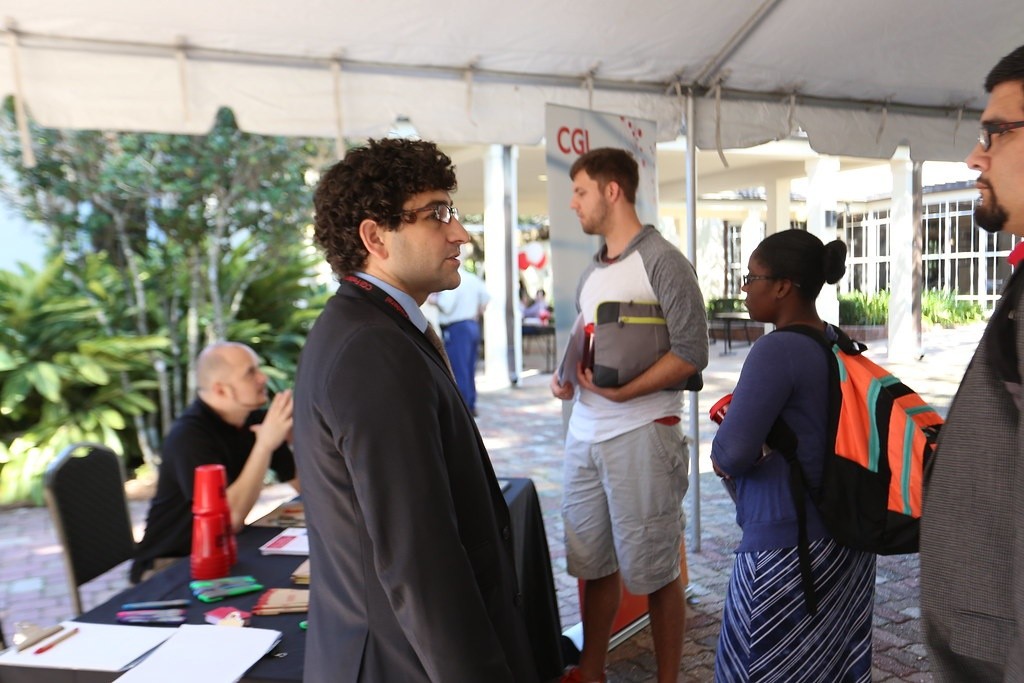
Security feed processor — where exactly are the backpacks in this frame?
[765,322,946,557]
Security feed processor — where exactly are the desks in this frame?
[521,324,558,374]
[73,475,567,683]
[711,311,751,355]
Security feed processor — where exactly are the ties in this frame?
[425,320,456,385]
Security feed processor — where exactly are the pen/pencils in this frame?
[121,599,192,610]
[116,616,188,624]
[18,625,64,652]
[115,609,187,615]
[252,588,309,615]
[189,575,264,603]
[35,628,79,654]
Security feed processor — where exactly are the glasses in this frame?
[393,202,459,224]
[977,120,1024,152]
[743,275,784,286]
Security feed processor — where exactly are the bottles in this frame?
[580,322,595,374]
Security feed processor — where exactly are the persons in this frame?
[550,147,709,683]
[919,46,1024,683]
[427,270,490,413]
[128,342,300,585]
[293,138,539,683]
[710,227,877,683]
[519,281,545,317]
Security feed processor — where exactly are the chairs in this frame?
[41,440,137,618]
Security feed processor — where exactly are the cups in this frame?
[190,465,238,579]
[710,394,772,461]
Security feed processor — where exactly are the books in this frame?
[259,526,310,556]
